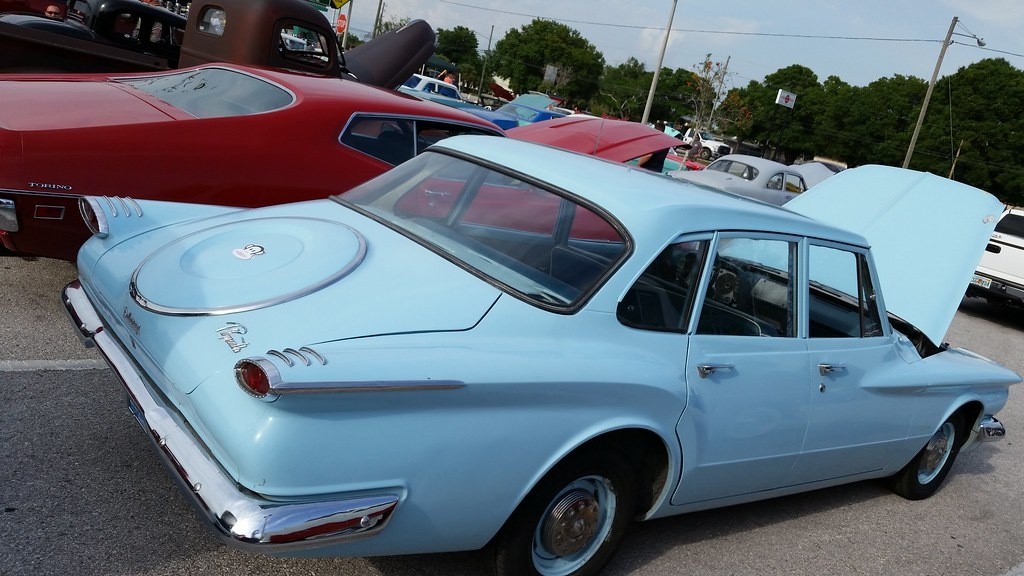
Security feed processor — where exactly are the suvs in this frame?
[965,209,1023,308]
[0,0,734,173]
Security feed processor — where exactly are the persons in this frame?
[43,4,63,21]
[572,106,580,114]
[443,69,459,89]
[685,133,704,171]
[291,30,309,50]
[637,122,670,174]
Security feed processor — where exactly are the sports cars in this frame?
[1,62,694,255]
[665,154,835,207]
[63,133,1023,575]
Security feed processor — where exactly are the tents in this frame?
[421,53,461,93]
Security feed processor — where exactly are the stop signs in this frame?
[337,14,347,34]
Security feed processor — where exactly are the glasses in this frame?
[45,10,61,15]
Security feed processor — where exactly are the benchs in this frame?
[378,131,432,165]
[411,216,580,302]
[549,245,776,339]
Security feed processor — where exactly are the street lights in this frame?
[902,17,986,169]
